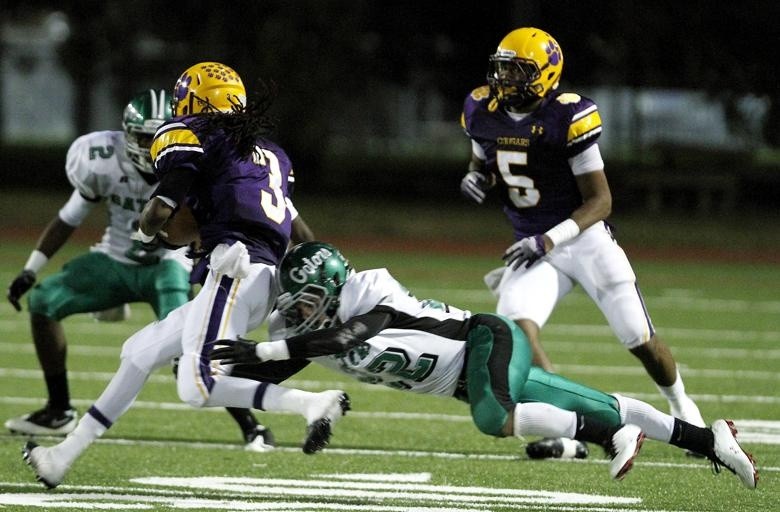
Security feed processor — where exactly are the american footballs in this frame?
[156,203,199,246]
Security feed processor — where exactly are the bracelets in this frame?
[283,335,308,360]
[544,218,581,247]
[23,249,49,275]
[137,226,156,245]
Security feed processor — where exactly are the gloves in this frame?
[501,233,555,272]
[208,334,273,367]
[459,170,488,209]
[6,269,37,312]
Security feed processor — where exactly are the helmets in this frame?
[486,26,566,108]
[121,88,173,176]
[169,61,249,119]
[273,240,352,337]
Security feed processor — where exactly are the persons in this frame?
[461,27,711,460]
[4,88,276,453]
[203,239,759,491]
[22,61,351,491]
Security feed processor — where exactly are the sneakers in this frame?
[302,389,353,456]
[3,407,80,440]
[525,398,710,483]
[22,439,70,490]
[241,424,277,454]
[712,416,760,492]
